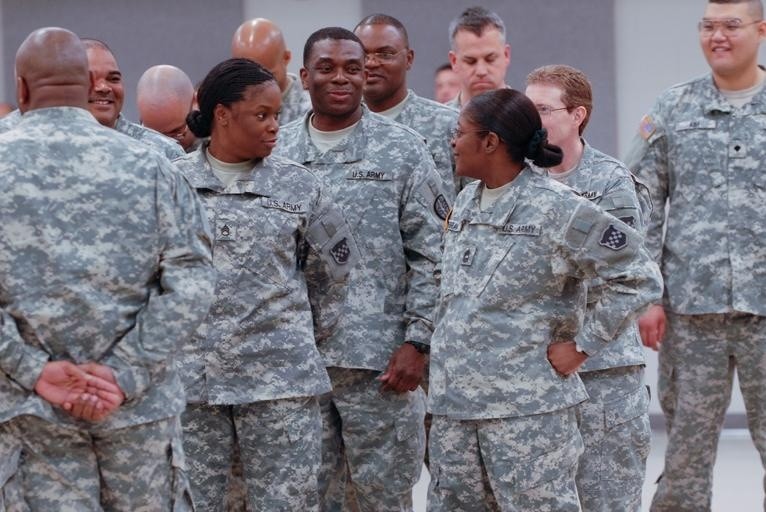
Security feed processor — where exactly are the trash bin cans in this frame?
[575,343,588,355]
[404,340,430,355]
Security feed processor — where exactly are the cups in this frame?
[450,125,491,140]
[139,94,198,138]
[698,19,761,38]
[533,103,576,119]
[365,47,407,66]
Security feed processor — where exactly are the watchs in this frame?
[404,340,430,355]
[575,343,588,355]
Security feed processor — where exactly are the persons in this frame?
[423,87,665,510]
[0,28,216,511]
[136,65,209,154]
[79,38,186,160]
[523,65,654,509]
[443,7,512,110]
[433,64,463,103]
[351,14,481,204]
[232,18,314,126]
[274,28,455,510]
[165,58,361,510]
[628,0,766,512]
